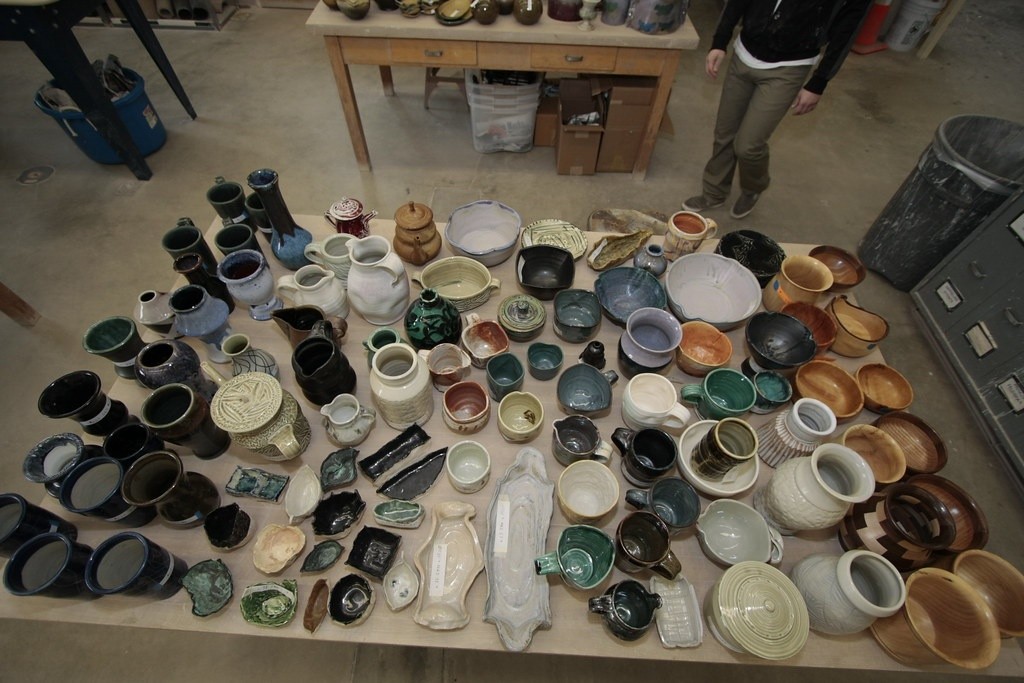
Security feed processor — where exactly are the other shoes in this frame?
[731,191,761,218]
[682,195,726,212]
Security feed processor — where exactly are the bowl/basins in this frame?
[412,196,602,343]
[870,473,1024,671]
[706,559,807,660]
[598,229,891,378]
[795,360,949,483]
[698,497,785,570]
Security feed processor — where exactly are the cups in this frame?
[664,212,717,263]
[589,579,662,640]
[0,492,79,559]
[417,313,562,443]
[87,531,188,603]
[629,477,700,534]
[610,426,679,481]
[617,510,681,581]
[680,367,757,420]
[537,524,616,592]
[556,362,619,418]
[552,412,613,464]
[621,373,692,429]
[4,534,106,601]
[446,439,491,494]
[692,419,761,483]
[752,366,792,414]
[556,459,622,523]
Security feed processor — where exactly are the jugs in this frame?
[292,319,357,406]
[321,195,377,240]
[277,262,350,324]
[317,393,375,448]
[346,234,412,327]
[362,326,413,368]
[303,233,366,281]
[199,361,314,462]
[389,201,441,263]
[272,304,349,351]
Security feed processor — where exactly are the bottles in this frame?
[160,167,315,377]
[18,311,224,526]
[403,289,463,351]
[756,396,837,467]
[133,290,186,339]
[753,442,876,535]
[367,343,433,429]
[833,484,958,573]
[790,548,907,637]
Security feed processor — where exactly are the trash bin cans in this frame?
[855,112,1024,292]
[33,55,169,165]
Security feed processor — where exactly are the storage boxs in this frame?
[534,73,675,175]
[464,67,548,155]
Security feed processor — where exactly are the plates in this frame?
[168,421,560,651]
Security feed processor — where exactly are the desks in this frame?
[304,1,700,181]
[0,209,1024,682]
[0,0,197,180]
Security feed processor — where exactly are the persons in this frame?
[681,0,877,219]
